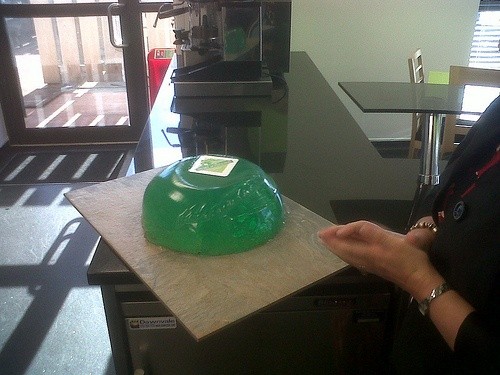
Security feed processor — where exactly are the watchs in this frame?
[417,282,452,316]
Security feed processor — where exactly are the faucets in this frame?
[208,37,224,50]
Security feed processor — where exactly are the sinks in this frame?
[173,56,273,98]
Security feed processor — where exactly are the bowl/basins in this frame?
[141,153,285,258]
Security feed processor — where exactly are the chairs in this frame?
[407,48,500,159]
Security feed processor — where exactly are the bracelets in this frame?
[409,222,440,233]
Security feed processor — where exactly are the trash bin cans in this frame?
[148,47,176,109]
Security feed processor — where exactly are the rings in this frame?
[361,264,366,273]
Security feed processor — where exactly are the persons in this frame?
[157,50,164,58]
[318,95,500,375]
